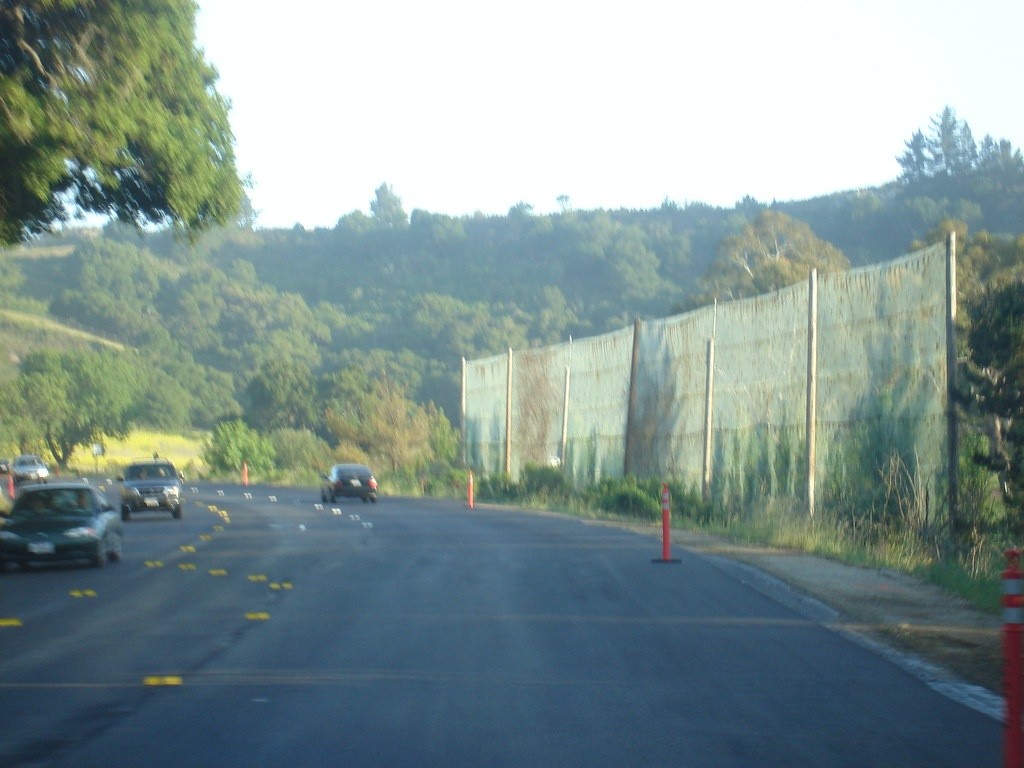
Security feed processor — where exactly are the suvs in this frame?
[116,462,182,522]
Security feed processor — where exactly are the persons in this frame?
[76,490,91,512]
[157,467,168,479]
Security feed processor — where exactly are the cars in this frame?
[12,455,49,486]
[0,481,123,574]
[319,464,377,504]
